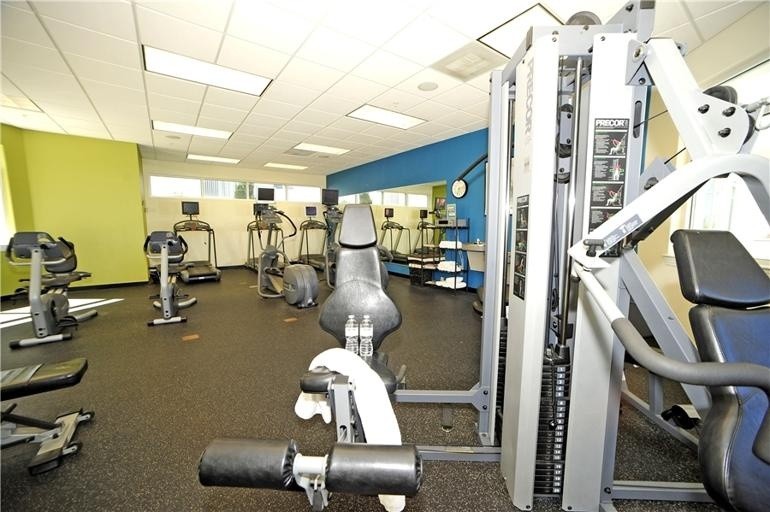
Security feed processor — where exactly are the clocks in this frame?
[451,179,468,198]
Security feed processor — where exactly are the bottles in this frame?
[359,313,373,357]
[345,314,358,355]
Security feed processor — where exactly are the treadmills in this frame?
[379,221,412,263]
[172,220,222,283]
[247,204,296,276]
[414,221,441,258]
[298,219,328,269]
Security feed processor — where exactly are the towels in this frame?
[293,346,409,512]
